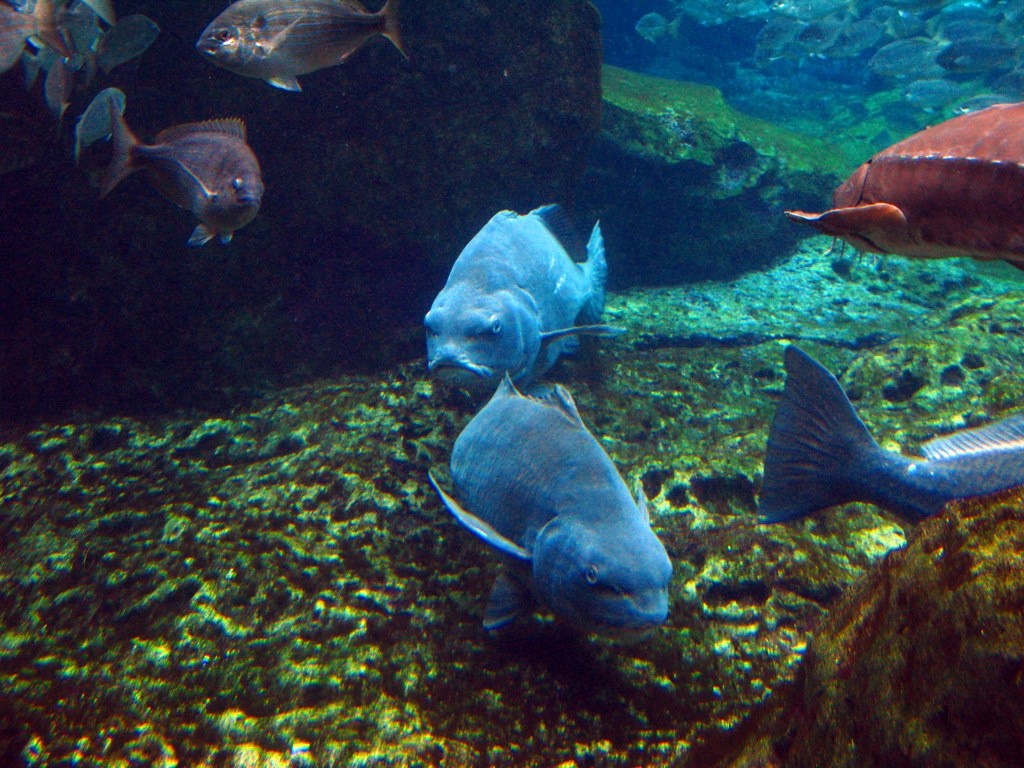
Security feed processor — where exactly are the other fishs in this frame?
[0,1,1024,634]
